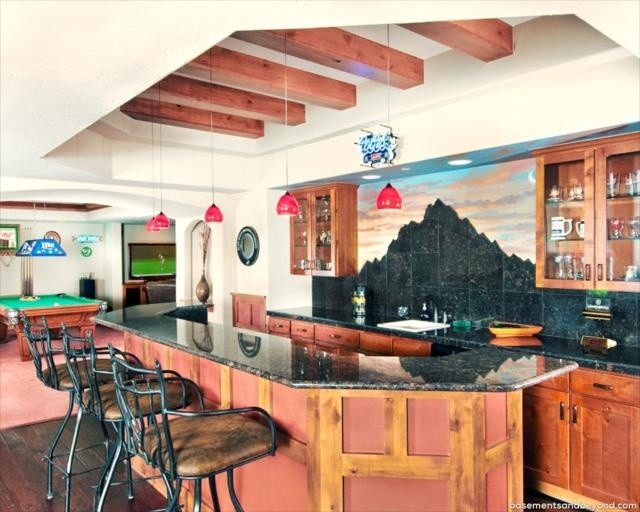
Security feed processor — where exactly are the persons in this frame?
[158,253,166,273]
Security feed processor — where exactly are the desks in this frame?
[122,282,146,308]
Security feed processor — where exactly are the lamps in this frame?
[277,30,298,215]
[158,82,170,227]
[15,238,38,256]
[205,48,223,221]
[144,86,158,232]
[30,239,65,257]
[376,25,403,210]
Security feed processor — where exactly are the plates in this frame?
[490,336,543,346]
[489,317,542,336]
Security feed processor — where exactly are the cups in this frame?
[608,217,639,237]
[552,216,572,239]
[553,254,583,278]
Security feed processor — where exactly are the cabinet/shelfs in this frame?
[312,324,359,356]
[523,365,639,510]
[269,314,314,343]
[359,329,433,362]
[290,183,360,277]
[528,133,640,294]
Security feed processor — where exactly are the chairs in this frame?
[13,314,143,510]
[97,343,281,512]
[56,326,187,512]
